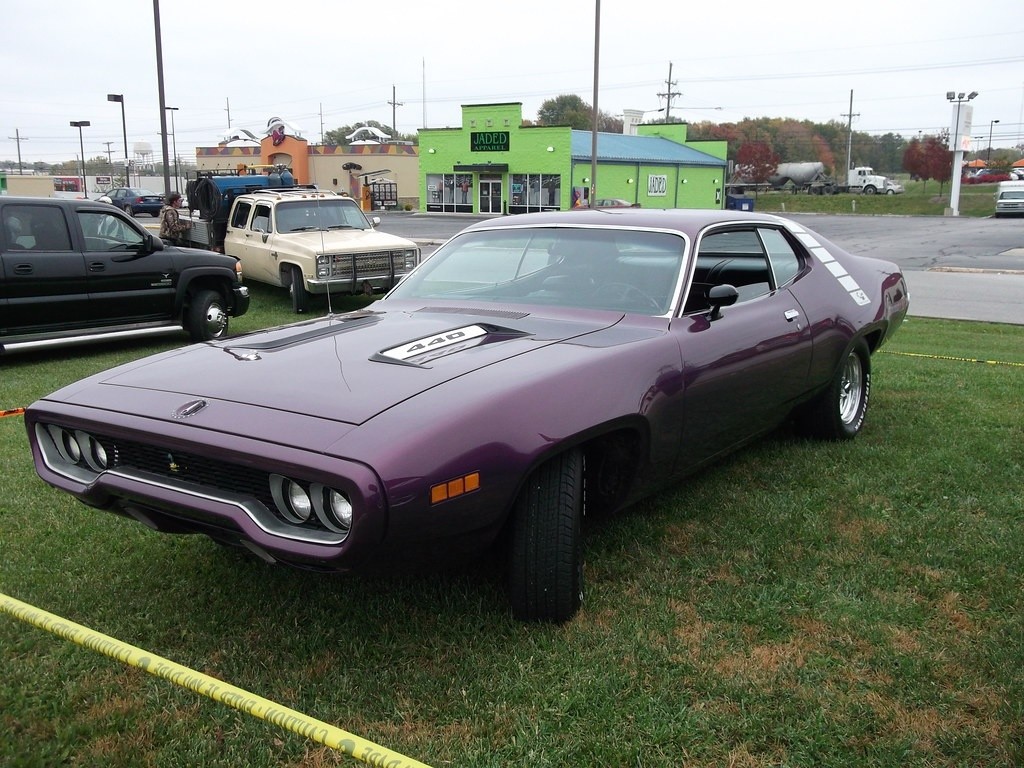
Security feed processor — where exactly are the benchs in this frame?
[612,253,800,312]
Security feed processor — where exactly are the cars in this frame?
[962,168,1024,218]
[885,180,905,196]
[574,198,633,209]
[105,187,165,219]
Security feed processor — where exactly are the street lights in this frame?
[69,120,91,199]
[164,107,179,195]
[107,94,130,188]
[945,91,980,206]
[987,120,1001,169]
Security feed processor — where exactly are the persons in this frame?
[98,196,123,240]
[461,181,468,203]
[534,180,541,205]
[159,194,190,246]
[524,237,656,312]
[521,180,527,204]
[548,179,555,205]
[439,180,443,203]
[448,180,454,203]
[65,179,79,191]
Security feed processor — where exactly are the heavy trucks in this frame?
[806,166,888,195]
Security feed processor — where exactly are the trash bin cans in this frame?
[727,193,754,212]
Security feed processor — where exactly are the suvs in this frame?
[0,192,252,355]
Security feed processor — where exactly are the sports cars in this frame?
[21,205,911,626]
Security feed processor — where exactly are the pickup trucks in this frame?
[175,186,422,314]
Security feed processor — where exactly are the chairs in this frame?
[28,215,70,250]
